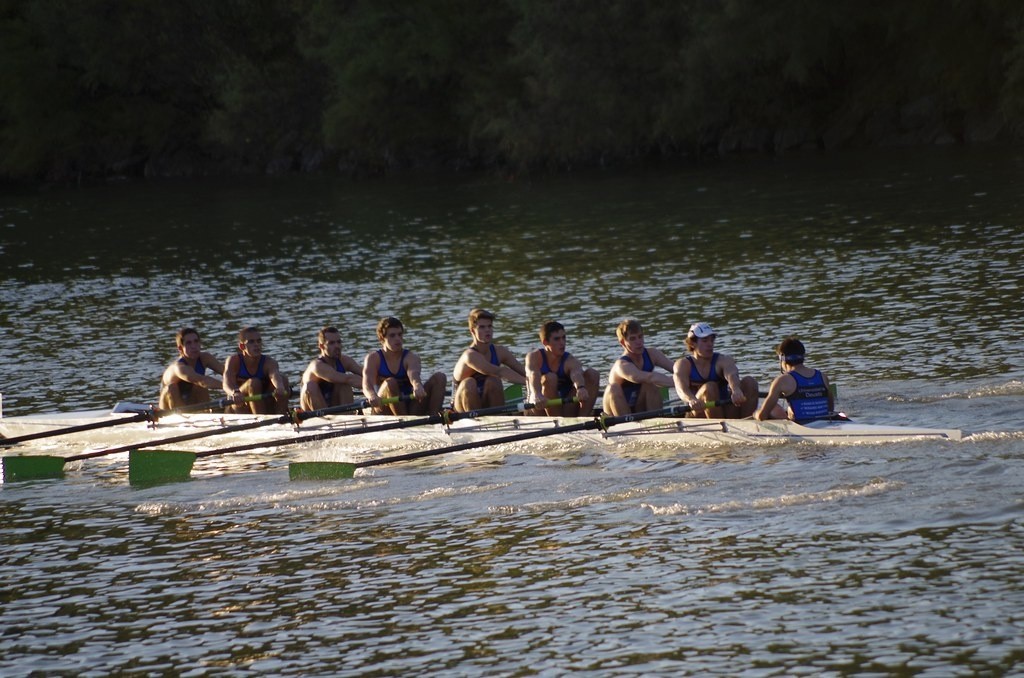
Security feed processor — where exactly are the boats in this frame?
[1,403,965,487]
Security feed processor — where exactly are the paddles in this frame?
[128,383,673,492]
[286,381,839,484]
[0,384,301,447]
[0,389,453,486]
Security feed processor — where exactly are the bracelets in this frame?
[575,385,585,389]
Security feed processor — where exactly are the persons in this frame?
[361,317,446,415]
[751,338,834,424]
[601,319,674,416]
[158,328,224,413]
[221,327,289,414]
[450,308,525,413]
[672,322,758,418]
[299,327,362,415]
[524,322,599,416]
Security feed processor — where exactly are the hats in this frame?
[686,321,718,338]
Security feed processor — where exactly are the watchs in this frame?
[231,389,240,396]
